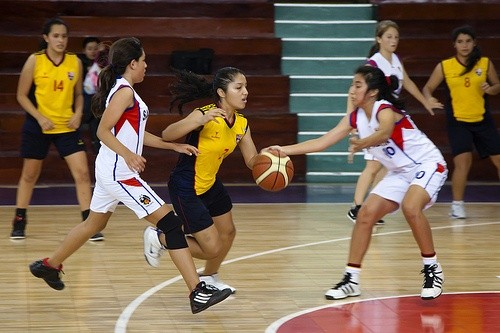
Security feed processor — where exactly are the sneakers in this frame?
[421,262,445,299]
[189,281,232,313]
[90,233,103,241]
[30,260,66,290]
[144,226,166,267]
[199,273,236,294]
[10,215,26,238]
[375,220,384,224]
[449,206,465,218]
[347,210,358,222]
[324,273,360,299]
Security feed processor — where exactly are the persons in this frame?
[260,65,448,299]
[79,34,101,154]
[29,37,232,314]
[10,18,104,240]
[144,66,259,294]
[347,20,444,232]
[423,28,500,219]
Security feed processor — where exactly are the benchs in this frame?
[0,0,500,205]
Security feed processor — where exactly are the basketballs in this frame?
[253,149,294,192]
